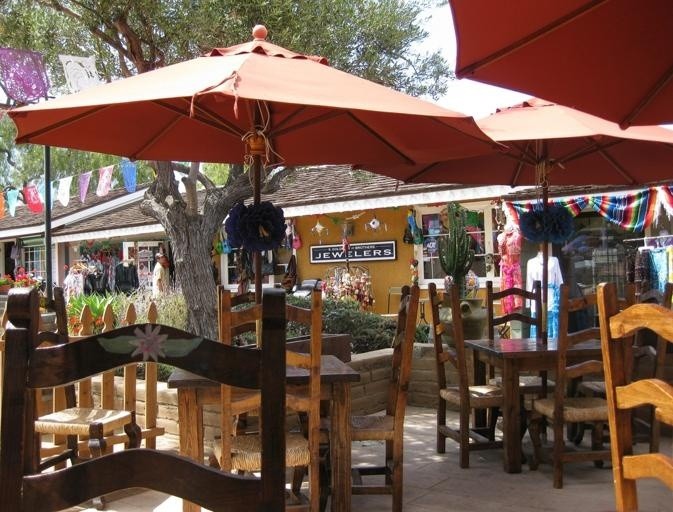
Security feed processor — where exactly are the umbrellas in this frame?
[1,24,514,357]
[347,90,671,348]
[445,1,672,133]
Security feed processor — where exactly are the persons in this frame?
[560,224,673,331]
[153,254,169,299]
[524,242,563,339]
[497,198,523,313]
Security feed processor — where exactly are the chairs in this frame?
[214,282,330,511]
[428,281,504,470]
[526,281,638,490]
[482,279,557,441]
[320,283,421,510]
[2,283,287,511]
[575,277,671,512]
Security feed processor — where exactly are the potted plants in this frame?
[434,200,490,349]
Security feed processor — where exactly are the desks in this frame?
[164,353,364,511]
[462,333,603,476]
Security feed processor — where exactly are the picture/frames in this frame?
[309,239,399,264]
